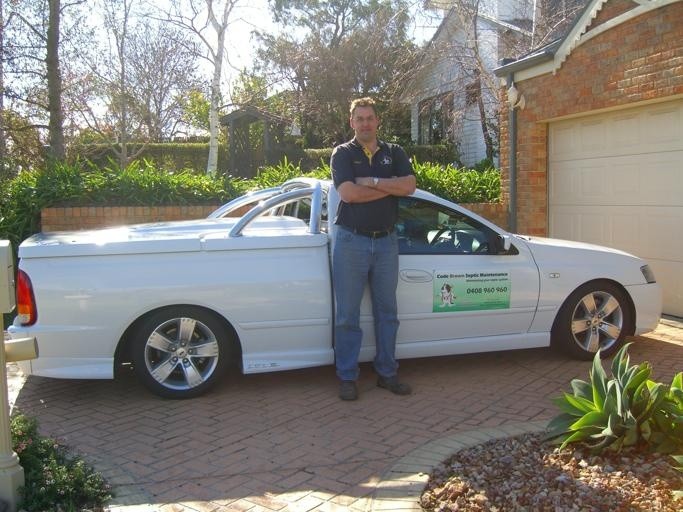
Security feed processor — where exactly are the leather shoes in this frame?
[340,382,356,400]
[376,376,410,394]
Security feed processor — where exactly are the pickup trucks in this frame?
[6,177,661,401]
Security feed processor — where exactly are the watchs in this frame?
[372,176,380,188]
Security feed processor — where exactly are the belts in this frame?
[357,223,394,237]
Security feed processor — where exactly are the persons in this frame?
[330,97,416,400]
